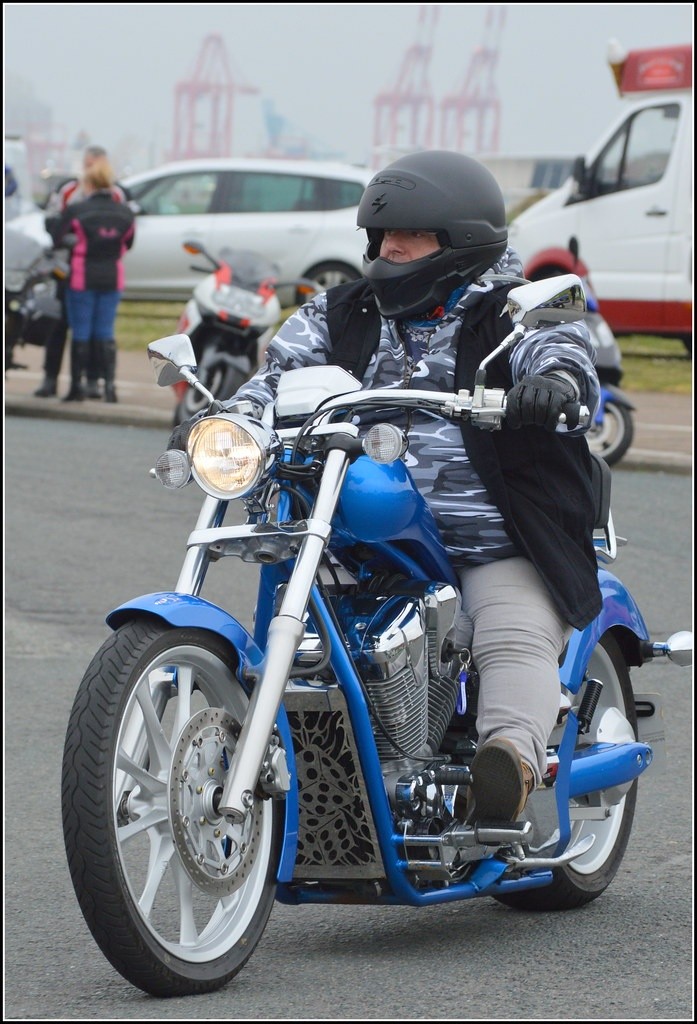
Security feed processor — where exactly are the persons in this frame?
[44,156,137,404]
[33,147,131,399]
[169,151,600,829]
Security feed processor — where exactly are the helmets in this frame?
[357,151,508,321]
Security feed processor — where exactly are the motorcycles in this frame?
[61,273,697,997]
[159,238,329,432]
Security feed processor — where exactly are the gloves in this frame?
[507,373,580,433]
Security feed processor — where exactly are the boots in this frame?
[61,341,89,401]
[100,341,120,403]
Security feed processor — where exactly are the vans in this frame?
[491,46,692,357]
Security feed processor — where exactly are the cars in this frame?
[9,157,384,309]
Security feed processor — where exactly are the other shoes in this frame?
[467,739,533,825]
[85,383,102,399]
[34,379,57,397]
